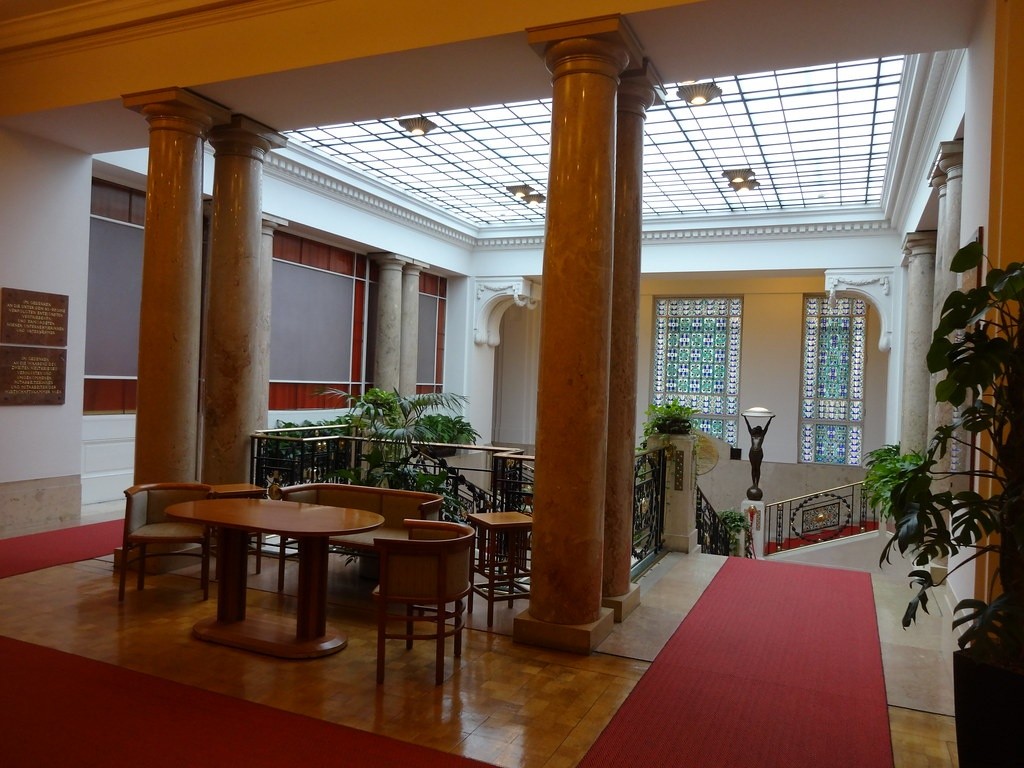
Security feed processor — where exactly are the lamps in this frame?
[399,117,437,136]
[721,170,760,194]
[506,184,546,204]
[676,83,722,105]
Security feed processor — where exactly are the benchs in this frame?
[279,483,444,591]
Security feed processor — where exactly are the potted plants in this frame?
[879,222,1024,768]
[643,398,699,463]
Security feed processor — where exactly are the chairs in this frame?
[118,482,214,600]
[374,519,475,686]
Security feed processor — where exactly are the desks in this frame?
[468,511,533,627]
[165,499,384,659]
[210,482,267,580]
